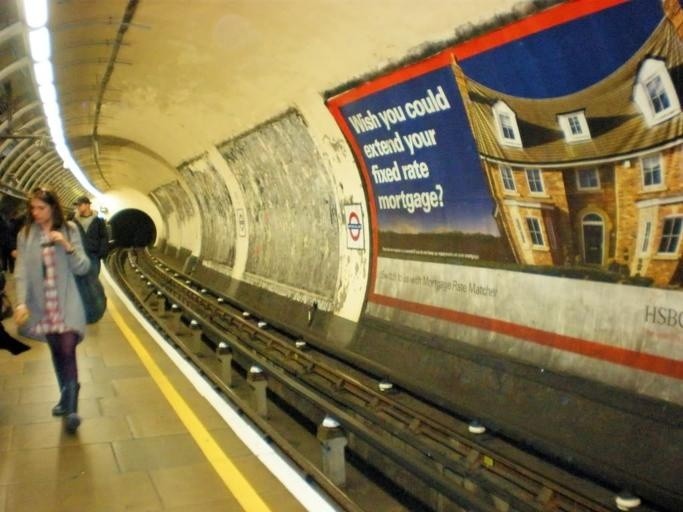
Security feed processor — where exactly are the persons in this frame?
[0,187,108,433]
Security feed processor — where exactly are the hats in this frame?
[72,196,90,204]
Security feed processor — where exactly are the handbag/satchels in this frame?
[74,269,107,324]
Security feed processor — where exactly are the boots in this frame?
[52,383,81,434]
